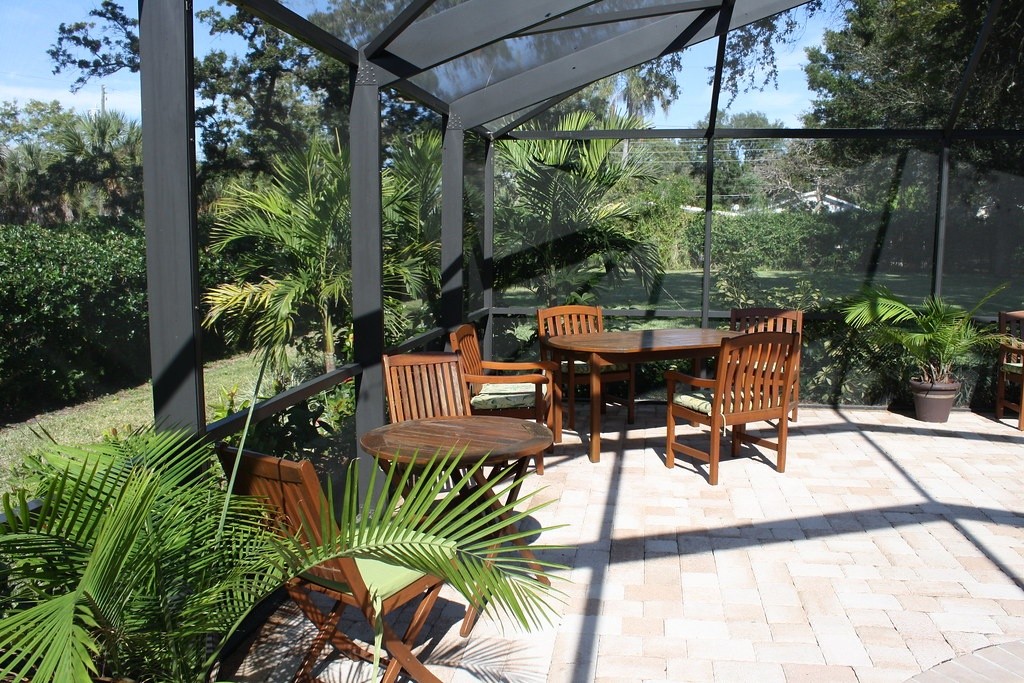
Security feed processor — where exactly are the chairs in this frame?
[382,305,635,516]
[663,308,803,485]
[995,309,1024,431]
[214,441,460,683]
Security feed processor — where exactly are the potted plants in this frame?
[840,280,1014,423]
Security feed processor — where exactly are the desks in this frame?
[360,416,554,637]
[546,329,745,463]
